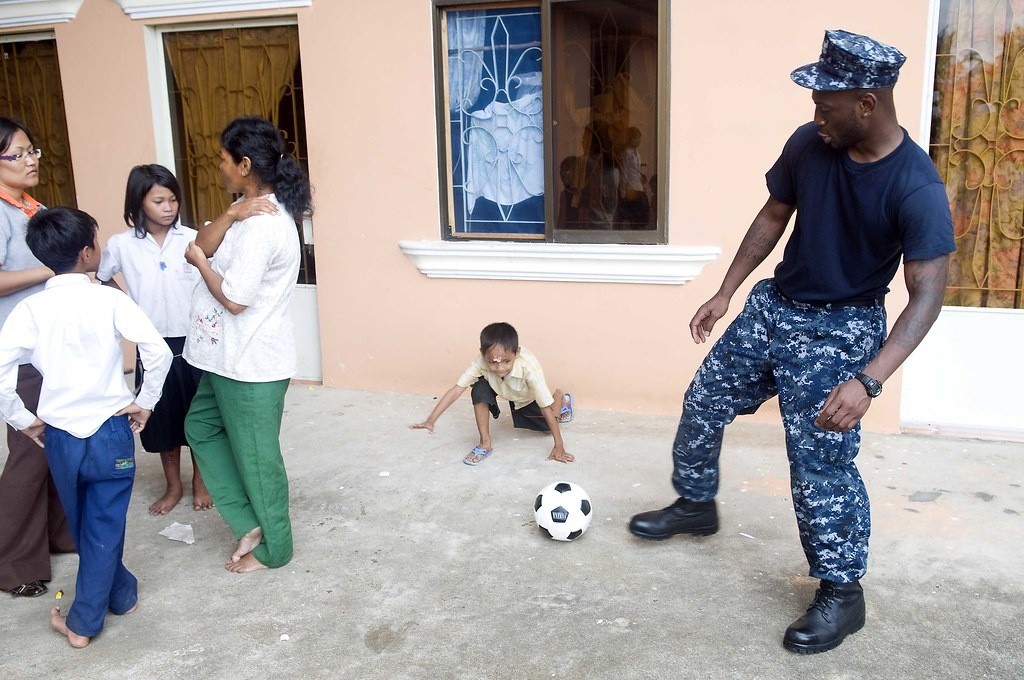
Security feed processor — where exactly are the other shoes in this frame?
[1,580,49,597]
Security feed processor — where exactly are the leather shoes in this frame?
[784,577,865,654]
[629,497,720,541]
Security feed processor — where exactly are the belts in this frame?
[805,296,886,309]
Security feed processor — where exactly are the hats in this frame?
[791,29,907,91]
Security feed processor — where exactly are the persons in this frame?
[94,164,217,516]
[553,120,657,230]
[1,205,173,648]
[409,323,575,465]
[0,115,79,597]
[183,117,312,572]
[630,31,956,656]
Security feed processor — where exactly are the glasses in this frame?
[0,149,42,161]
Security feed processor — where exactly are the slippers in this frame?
[463,445,494,466]
[557,392,573,423]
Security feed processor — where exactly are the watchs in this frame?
[854,373,882,398]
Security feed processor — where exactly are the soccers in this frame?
[532,479,594,544]
